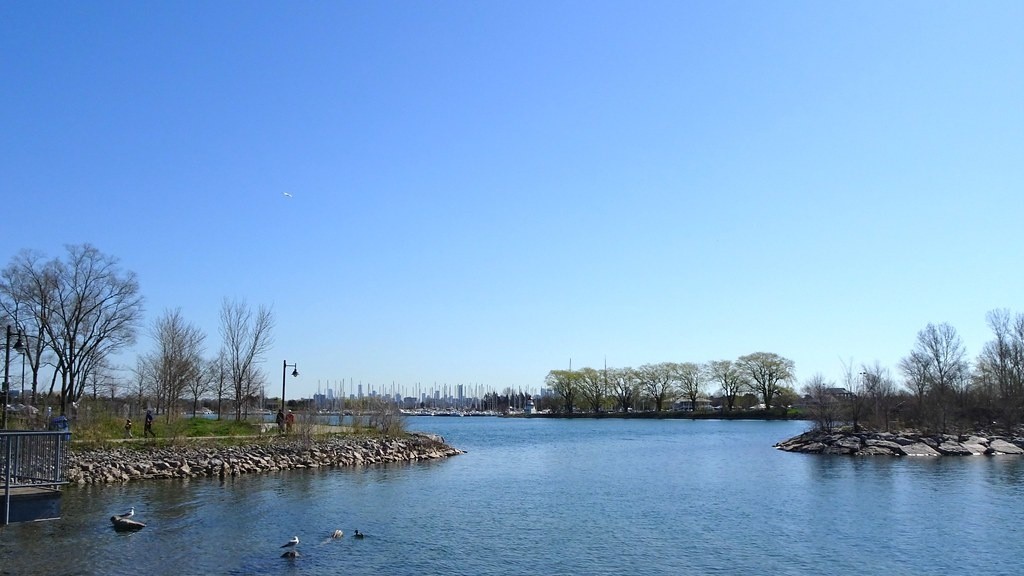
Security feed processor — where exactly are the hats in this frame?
[288,410,292,413]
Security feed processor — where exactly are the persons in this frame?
[276,409,294,432]
[144,409,156,438]
[123,419,133,439]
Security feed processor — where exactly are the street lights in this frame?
[282,359,300,414]
[1,325,27,429]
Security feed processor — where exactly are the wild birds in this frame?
[111,507,147,528]
[354,529,364,537]
[332,530,343,538]
[289,536,299,545]
[281,550,299,559]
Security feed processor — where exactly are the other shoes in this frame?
[123,437,126,439]
[152,434,156,438]
[129,436,133,439]
[145,436,147,438]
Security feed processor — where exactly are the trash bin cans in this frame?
[51,415,69,441]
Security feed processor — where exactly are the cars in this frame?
[6,403,41,416]
